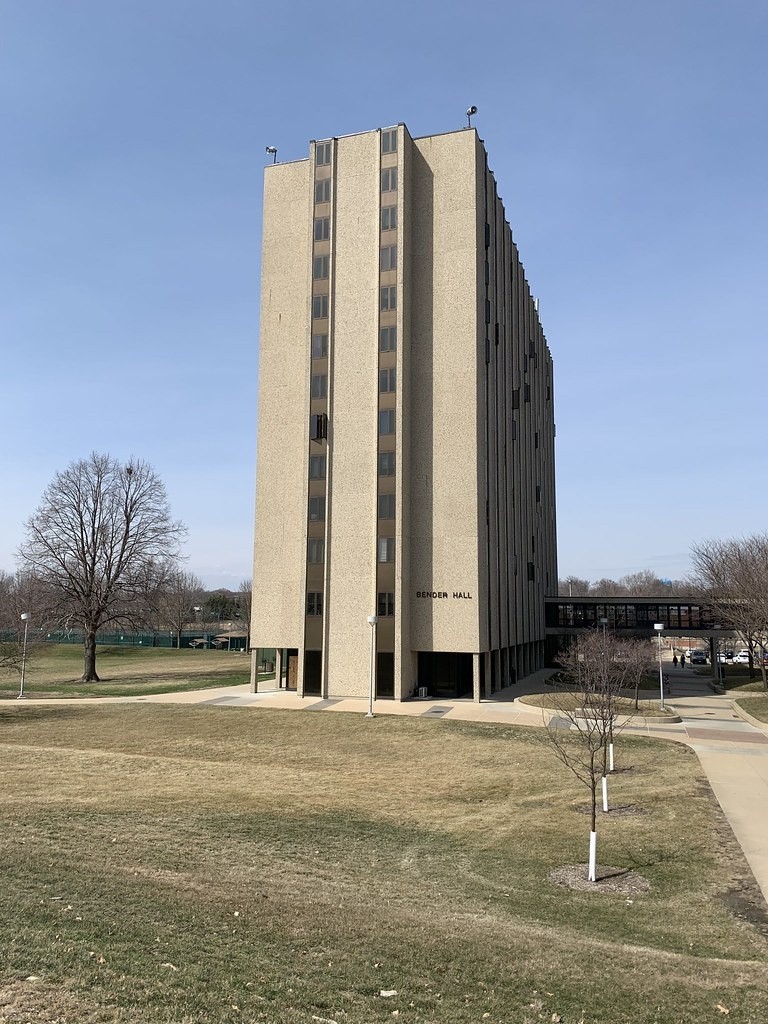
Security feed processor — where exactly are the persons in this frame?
[673,654,678,668]
[680,653,685,668]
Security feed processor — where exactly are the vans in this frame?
[717,654,727,663]
[733,652,750,664]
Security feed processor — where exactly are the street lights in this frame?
[365,615,379,718]
[653,624,664,711]
[17,612,32,699]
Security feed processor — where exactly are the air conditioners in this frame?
[419,687,427,698]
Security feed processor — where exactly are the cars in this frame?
[724,650,733,659]
[705,651,709,658]
[762,654,768,666]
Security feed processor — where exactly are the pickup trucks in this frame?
[690,651,706,664]
[686,651,691,657]
